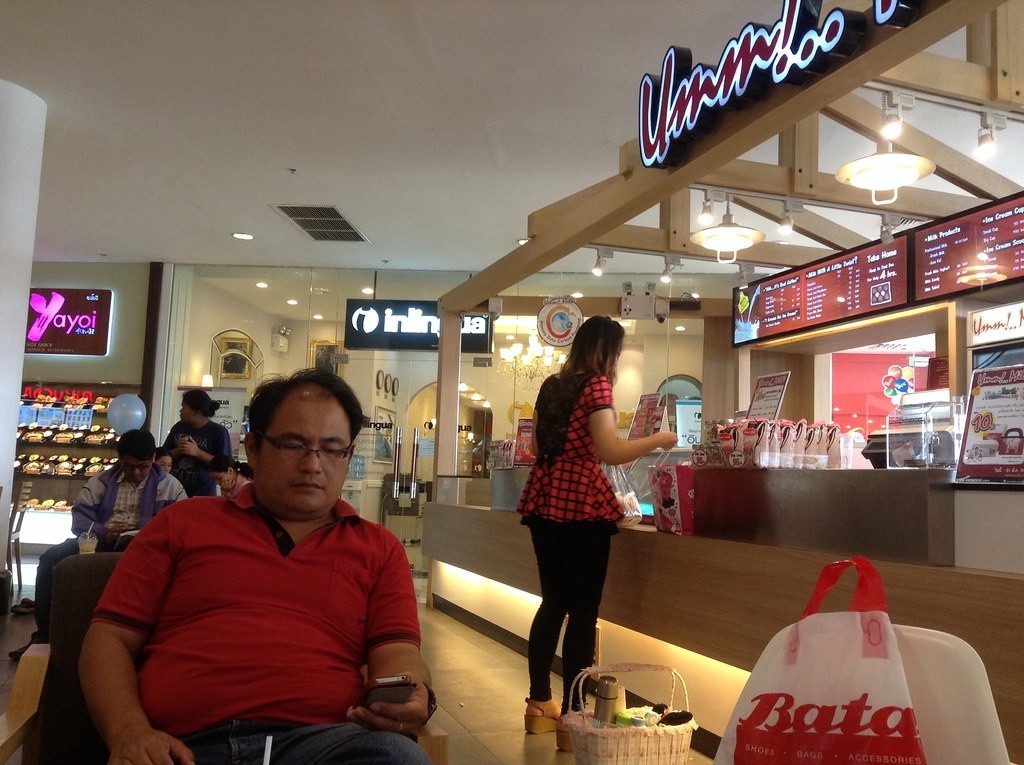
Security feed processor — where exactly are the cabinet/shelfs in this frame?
[11,382,141,511]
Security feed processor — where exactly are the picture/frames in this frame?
[220,337,253,379]
[311,339,344,378]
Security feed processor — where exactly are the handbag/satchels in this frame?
[647,445,696,537]
[713,555,927,765]
[599,458,643,528]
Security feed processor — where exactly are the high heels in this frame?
[556,716,573,752]
[524,697,563,735]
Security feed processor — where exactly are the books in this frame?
[114,530,140,550]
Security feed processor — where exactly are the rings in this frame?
[400,723,402,731]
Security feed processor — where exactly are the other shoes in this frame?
[10,598,35,613]
[8,632,48,659]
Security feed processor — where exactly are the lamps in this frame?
[619,281,657,319]
[835,139,936,205]
[689,189,766,264]
[499,334,567,390]
[660,264,673,283]
[699,189,725,225]
[778,202,805,233]
[977,113,1007,154]
[592,257,606,276]
[272,326,291,352]
[881,92,916,139]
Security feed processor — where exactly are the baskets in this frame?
[561,660,699,765]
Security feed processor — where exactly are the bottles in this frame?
[615,712,662,728]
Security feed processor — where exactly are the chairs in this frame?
[7,481,33,594]
[0,552,450,765]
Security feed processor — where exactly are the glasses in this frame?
[256,432,355,464]
[120,455,152,471]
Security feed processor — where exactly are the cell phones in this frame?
[357,675,413,710]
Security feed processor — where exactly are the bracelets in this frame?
[423,682,438,726]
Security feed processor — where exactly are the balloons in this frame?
[107,394,146,436]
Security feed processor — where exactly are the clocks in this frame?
[377,370,400,395]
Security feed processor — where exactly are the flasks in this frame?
[594,675,620,723]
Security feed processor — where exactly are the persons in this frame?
[8,429,189,658]
[156,447,173,474]
[163,389,232,497]
[78,367,438,765]
[206,454,254,497]
[516,316,679,751]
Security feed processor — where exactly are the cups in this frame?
[78,532,99,554]
